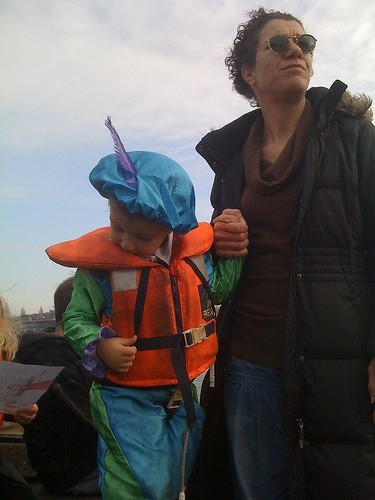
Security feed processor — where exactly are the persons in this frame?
[0,283,39,500]
[45,116,245,500]
[16,277,102,495]
[184,6,375,500]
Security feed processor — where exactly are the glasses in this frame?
[264,33,317,55]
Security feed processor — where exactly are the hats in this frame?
[88,151,199,235]
